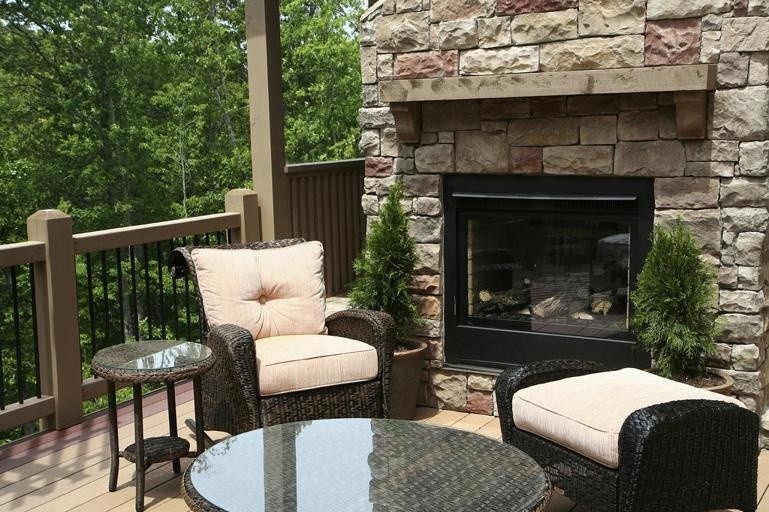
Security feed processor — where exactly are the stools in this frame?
[493,360,759,512]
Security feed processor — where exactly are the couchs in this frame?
[169,236,398,447]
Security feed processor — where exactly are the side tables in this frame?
[91,339,216,508]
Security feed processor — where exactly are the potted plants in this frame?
[630,215,733,398]
[349,178,427,420]
